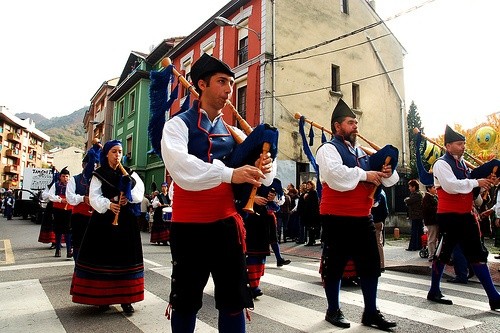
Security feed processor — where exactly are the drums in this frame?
[162,207,172,222]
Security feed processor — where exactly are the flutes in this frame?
[293,111,399,200]
[74,138,134,226]
[412,126,500,200]
[148,57,279,214]
[48,163,69,211]
[261,178,283,212]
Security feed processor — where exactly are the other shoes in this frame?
[488,296,500,310]
[296,240,304,244]
[67,248,73,258]
[446,275,470,284]
[121,303,134,313]
[304,242,315,246]
[427,292,453,304]
[49,245,55,249]
[277,258,291,266]
[361,309,397,328]
[250,287,263,300]
[55,249,62,257]
[325,308,351,328]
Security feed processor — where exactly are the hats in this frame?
[444,124,466,146]
[331,98,356,118]
[101,139,122,156]
[83,147,100,163]
[60,166,70,174]
[189,52,236,88]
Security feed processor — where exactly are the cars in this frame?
[13,187,51,226]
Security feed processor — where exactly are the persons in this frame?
[160,51,274,333]
[0,99,500,328]
